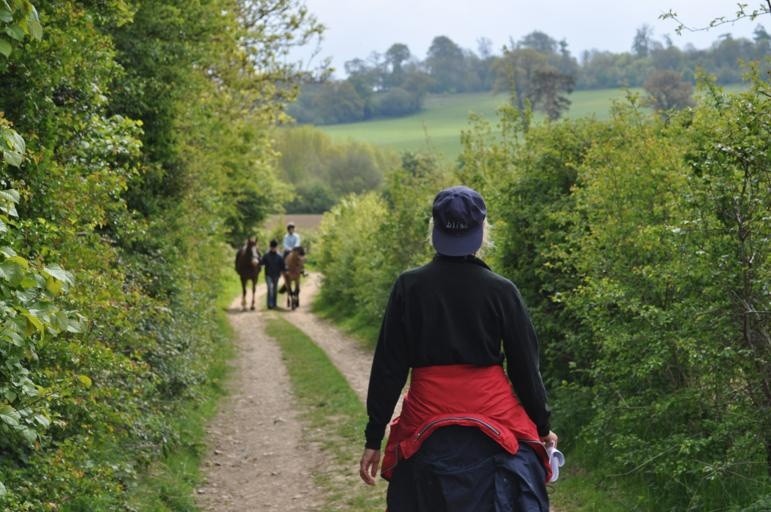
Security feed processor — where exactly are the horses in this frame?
[278,246,306,310]
[235,235,262,312]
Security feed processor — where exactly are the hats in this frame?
[431,185,487,258]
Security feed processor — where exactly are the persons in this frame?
[282,221,309,278]
[258,238,285,311]
[358,185,564,511]
